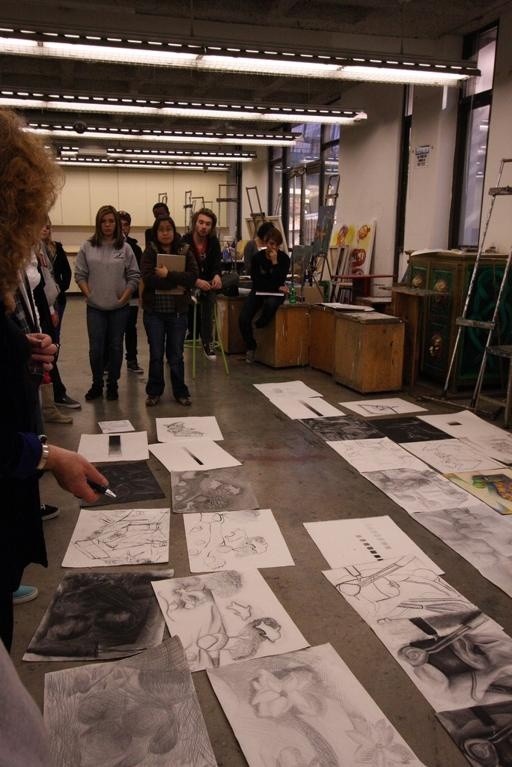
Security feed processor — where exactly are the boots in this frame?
[37,382,74,424]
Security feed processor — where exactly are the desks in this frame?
[220,291,406,395]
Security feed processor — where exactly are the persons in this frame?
[337,225,348,245]
[11,203,291,425]
[353,269,363,275]
[352,248,365,268]
[2,108,113,653]
[357,225,371,242]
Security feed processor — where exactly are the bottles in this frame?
[288,281,297,304]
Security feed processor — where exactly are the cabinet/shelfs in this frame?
[329,274,395,306]
[44,164,227,227]
[403,249,512,395]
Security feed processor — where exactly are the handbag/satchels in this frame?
[221,271,240,296]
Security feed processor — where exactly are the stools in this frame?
[471,343,511,429]
[183,293,232,381]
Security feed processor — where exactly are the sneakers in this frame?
[12,585,40,604]
[41,503,61,521]
[247,348,256,364]
[185,332,202,343]
[107,384,120,403]
[204,343,217,362]
[85,383,104,400]
[144,392,162,407]
[126,361,144,374]
[177,396,192,405]
[55,395,83,408]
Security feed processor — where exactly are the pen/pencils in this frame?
[86,475,119,501]
